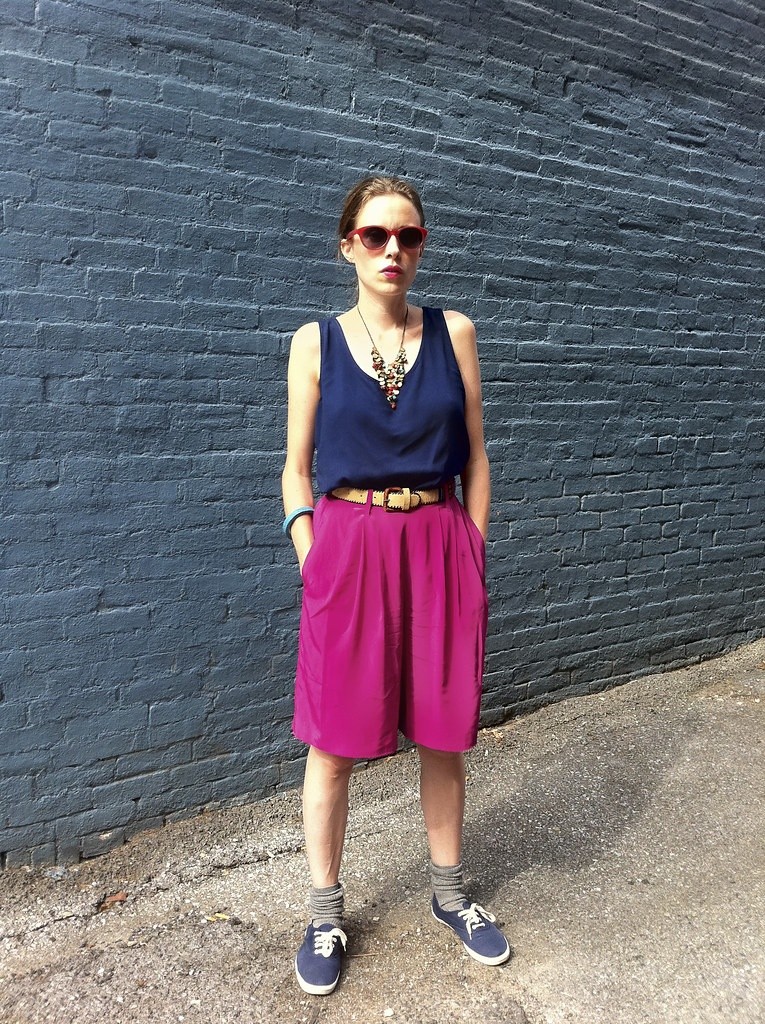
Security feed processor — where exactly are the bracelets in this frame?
[282,505,315,539]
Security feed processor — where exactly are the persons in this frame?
[280,175,509,995]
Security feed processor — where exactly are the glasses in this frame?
[346,226,429,253]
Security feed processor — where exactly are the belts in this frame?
[330,478,455,513]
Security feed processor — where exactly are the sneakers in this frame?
[296,922,347,995]
[435,890,511,965]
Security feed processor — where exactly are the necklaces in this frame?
[355,305,409,408]
